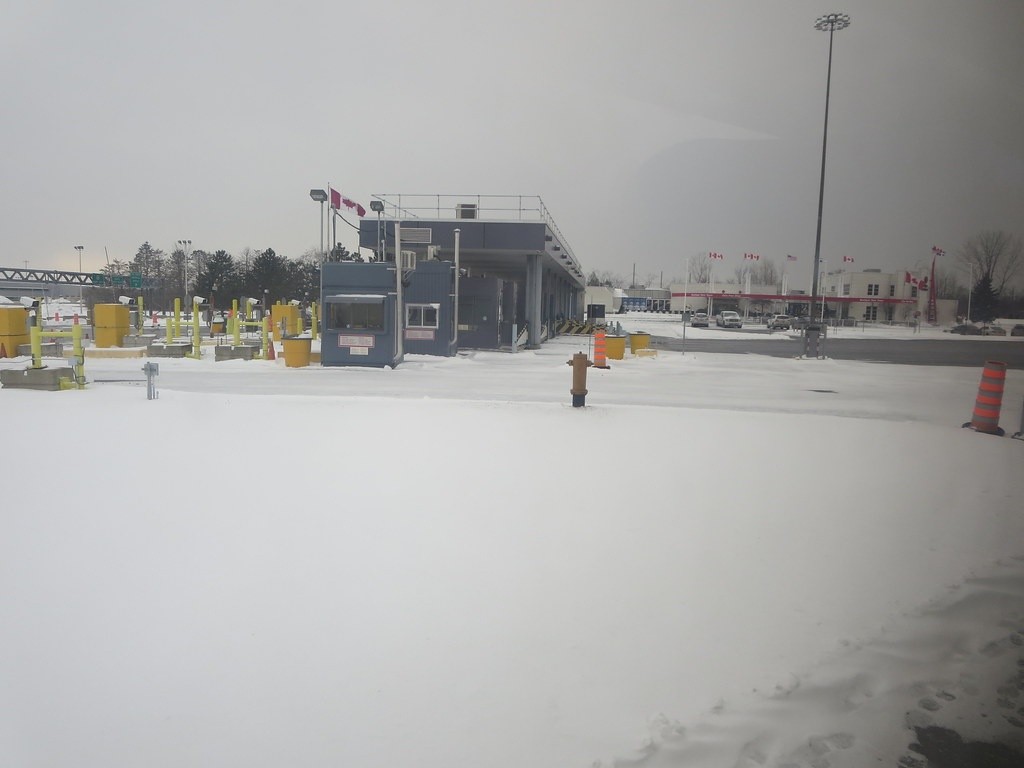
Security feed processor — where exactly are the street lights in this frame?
[966,263,973,324]
[807,12,852,351]
[178,239,192,293]
[310,189,328,331]
[819,259,828,322]
[370,200,385,262]
[75,246,84,314]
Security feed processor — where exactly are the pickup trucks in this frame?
[716,310,742,328]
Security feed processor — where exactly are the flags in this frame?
[708,252,723,260]
[904,270,925,290]
[787,254,796,262]
[329,187,367,217]
[844,256,854,263]
[744,253,760,261]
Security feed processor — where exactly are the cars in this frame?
[306,310,313,325]
[981,326,1006,336]
[950,325,979,335]
[77,299,84,303]
[56,298,71,304]
[1010,324,1024,337]
[791,316,818,331]
[690,313,708,326]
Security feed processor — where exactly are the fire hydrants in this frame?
[567,353,595,406]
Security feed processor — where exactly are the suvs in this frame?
[766,315,790,330]
